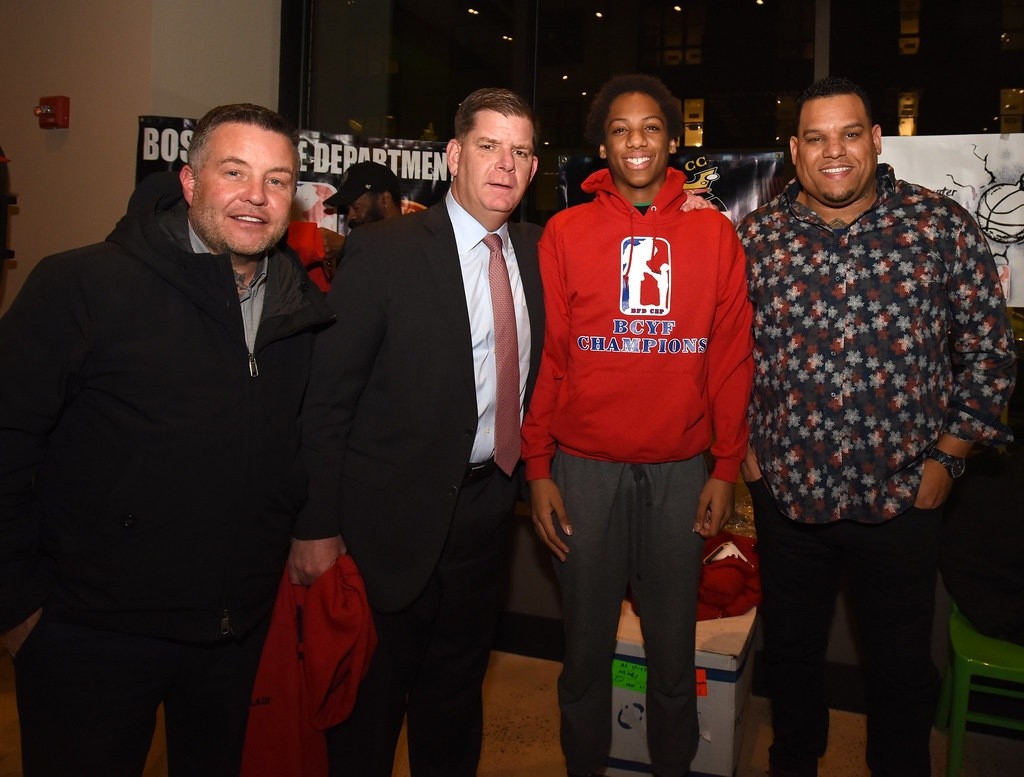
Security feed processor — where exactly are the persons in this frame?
[323,161,404,228]
[1,103,336,777]
[521,78,754,777]
[289,89,720,777]
[737,80,1012,777]
[287,223,348,296]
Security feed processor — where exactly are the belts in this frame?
[464,461,496,480]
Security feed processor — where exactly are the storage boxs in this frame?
[606,598,759,777]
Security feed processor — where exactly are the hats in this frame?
[324,161,398,209]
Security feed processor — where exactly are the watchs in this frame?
[928,449,966,479]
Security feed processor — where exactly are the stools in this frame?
[936,591,1024,777]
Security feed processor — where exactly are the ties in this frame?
[481,235,521,478]
